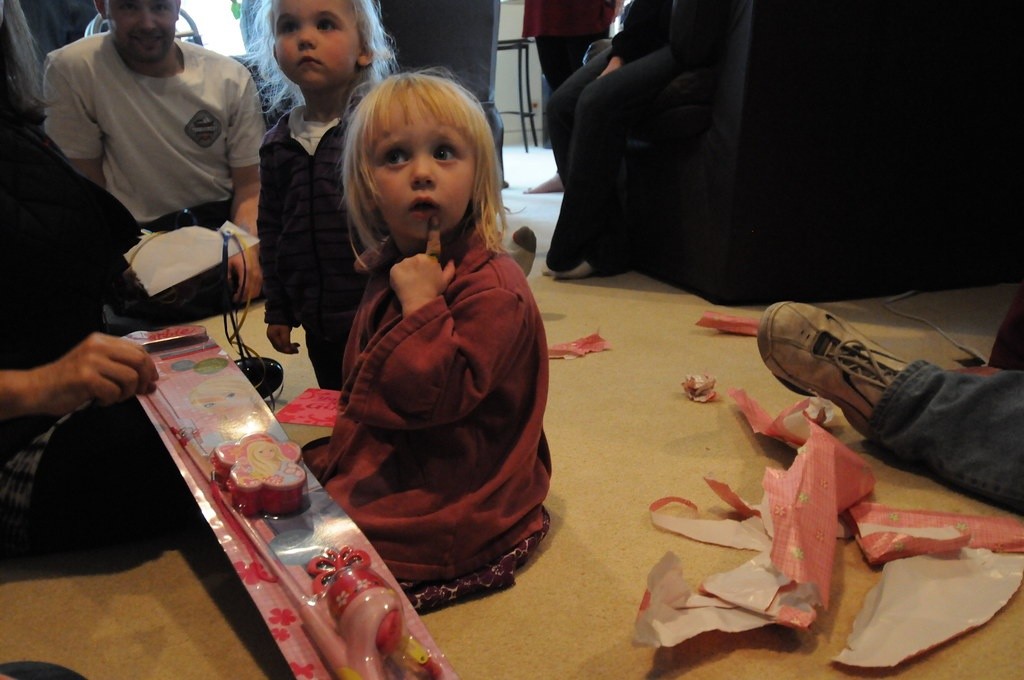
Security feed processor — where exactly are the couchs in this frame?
[581,0,1024,305]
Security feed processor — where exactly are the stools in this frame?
[495,38,538,154]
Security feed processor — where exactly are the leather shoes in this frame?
[235,357,283,399]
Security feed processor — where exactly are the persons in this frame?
[757,287,1024,517]
[42,0,264,324]
[0,0,285,553]
[256,0,396,397]
[518,0,627,90]
[543,0,733,279]
[375,0,509,188]
[297,72,554,613]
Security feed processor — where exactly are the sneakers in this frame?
[759,301,911,439]
